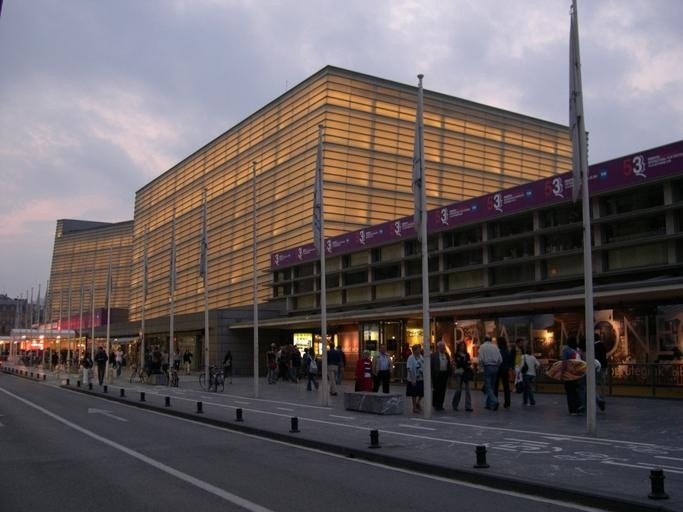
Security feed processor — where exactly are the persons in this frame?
[302,347,319,392]
[94,346,108,385]
[20,348,60,371]
[61,347,93,384]
[430,341,451,411]
[327,342,346,396]
[477,334,511,412]
[266,341,301,384]
[371,344,391,393]
[145,346,169,379]
[406,344,424,414]
[174,348,181,371]
[559,334,606,416]
[509,338,540,406]
[109,347,123,377]
[183,348,193,376]
[452,342,474,412]
[222,350,233,384]
[354,349,373,392]
[401,342,435,384]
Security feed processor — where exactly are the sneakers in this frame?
[522,400,536,406]
[452,403,475,412]
[433,402,445,412]
[485,401,510,411]
[307,382,338,396]
[413,403,422,412]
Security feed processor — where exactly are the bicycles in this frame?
[127,366,151,384]
[199,363,225,393]
[169,366,178,387]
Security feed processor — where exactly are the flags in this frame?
[197,205,208,289]
[167,216,177,296]
[309,142,326,261]
[566,7,587,211]
[142,237,148,305]
[14,262,115,330]
[409,89,425,244]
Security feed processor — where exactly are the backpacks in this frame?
[308,355,318,373]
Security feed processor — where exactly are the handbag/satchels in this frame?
[468,367,474,382]
[454,369,464,374]
[521,364,527,372]
[515,382,523,393]
